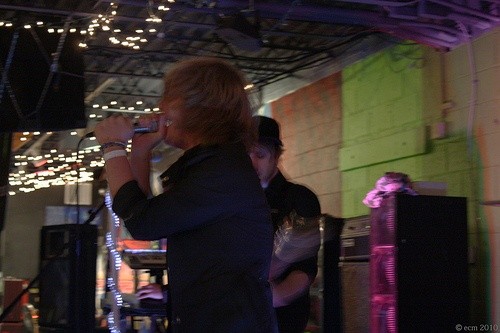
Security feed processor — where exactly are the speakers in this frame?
[368,193,471,333]
[38,225,97,331]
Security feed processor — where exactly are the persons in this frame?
[246,116,320,333]
[136,283,168,304]
[92,58,276,333]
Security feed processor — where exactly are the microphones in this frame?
[84,119,158,137]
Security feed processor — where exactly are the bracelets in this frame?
[100,141,127,161]
